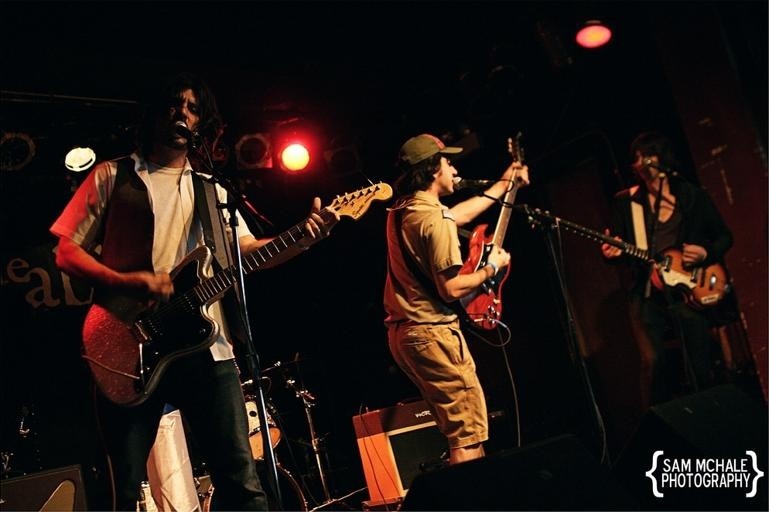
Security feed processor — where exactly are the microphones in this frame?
[452,175,495,192]
[173,120,200,141]
[642,156,679,176]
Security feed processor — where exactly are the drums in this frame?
[244,394,282,463]
[198,460,310,511]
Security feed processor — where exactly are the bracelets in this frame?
[487,261,501,276]
[298,241,312,252]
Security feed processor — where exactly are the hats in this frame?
[398,133,464,165]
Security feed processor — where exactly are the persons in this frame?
[382,134,531,468]
[599,137,734,409]
[48,70,344,512]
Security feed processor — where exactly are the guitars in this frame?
[81,178,393,409]
[455,132,524,330]
[527,208,731,312]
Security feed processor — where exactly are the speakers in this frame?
[0,463,88,512]
[609,382,768,509]
[397,430,641,510]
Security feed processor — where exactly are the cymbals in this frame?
[262,361,306,373]
